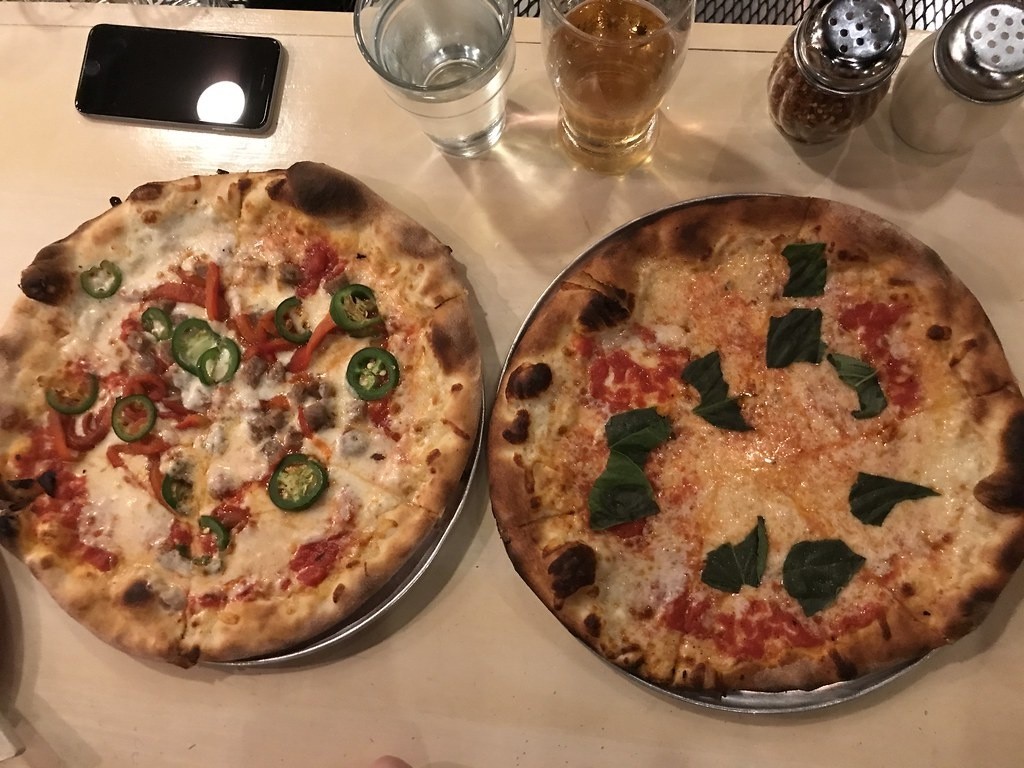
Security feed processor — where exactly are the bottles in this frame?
[891,0,1024,154]
[766,0,908,146]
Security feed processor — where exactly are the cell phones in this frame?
[74,23,283,134]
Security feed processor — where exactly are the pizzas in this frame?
[486,192,1024,698]
[0,162,483,667]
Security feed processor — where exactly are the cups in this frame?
[539,0,695,174]
[353,0,517,159]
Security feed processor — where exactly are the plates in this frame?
[485,191,1024,714]
[0,167,484,669]
